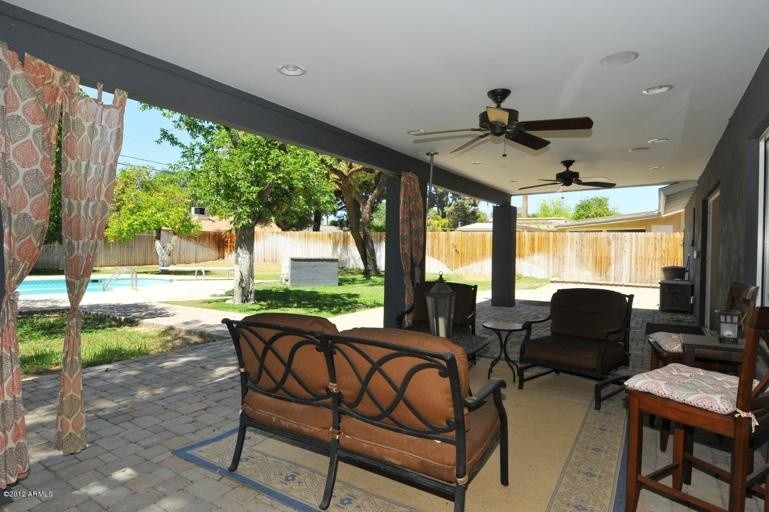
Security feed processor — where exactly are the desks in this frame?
[481,320,524,380]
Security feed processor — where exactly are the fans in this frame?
[516,160,617,192]
[404,87,594,154]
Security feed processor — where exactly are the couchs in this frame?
[219,312,513,512]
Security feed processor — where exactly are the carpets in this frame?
[168,353,627,512]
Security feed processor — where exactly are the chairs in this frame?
[621,282,768,511]
[517,289,634,412]
[397,283,478,365]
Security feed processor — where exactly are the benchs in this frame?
[158,263,235,280]
